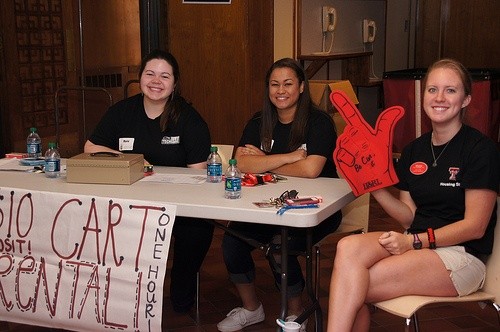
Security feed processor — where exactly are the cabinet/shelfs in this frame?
[385,0,500,76]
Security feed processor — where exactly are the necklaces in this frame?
[431,132,450,166]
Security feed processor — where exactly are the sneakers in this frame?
[216,301,265,332]
[282,314,308,332]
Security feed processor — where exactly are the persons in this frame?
[217,58,342,332]
[325,59,500,332]
[84,51,211,313]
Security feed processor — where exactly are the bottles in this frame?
[224,159,241,200]
[26,128,42,158]
[207,146,222,183]
[276,318,301,332]
[45,143,61,178]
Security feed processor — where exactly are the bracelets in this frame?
[427,228,436,249]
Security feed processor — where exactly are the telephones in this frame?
[323,6,337,32]
[364,19,376,43]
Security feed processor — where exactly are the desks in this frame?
[0,158,357,332]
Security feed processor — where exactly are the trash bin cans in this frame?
[383,67,500,154]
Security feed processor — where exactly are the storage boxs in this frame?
[308,79,359,112]
[66,152,145,185]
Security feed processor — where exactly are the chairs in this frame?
[368,197,500,332]
[297,117,370,302]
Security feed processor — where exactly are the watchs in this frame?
[413,233,422,250]
